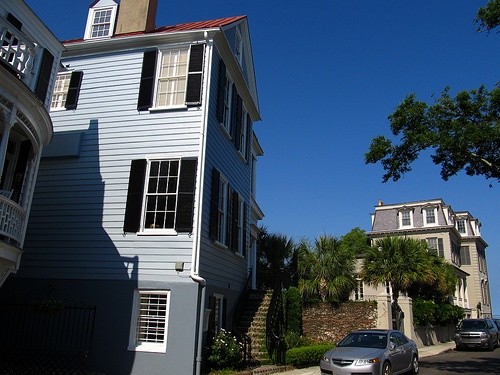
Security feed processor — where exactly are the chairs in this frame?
[359,336,371,345]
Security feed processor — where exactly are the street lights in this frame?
[191,274,206,375]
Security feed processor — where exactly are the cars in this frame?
[319,329,420,375]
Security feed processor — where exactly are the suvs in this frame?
[453,318,500,352]
